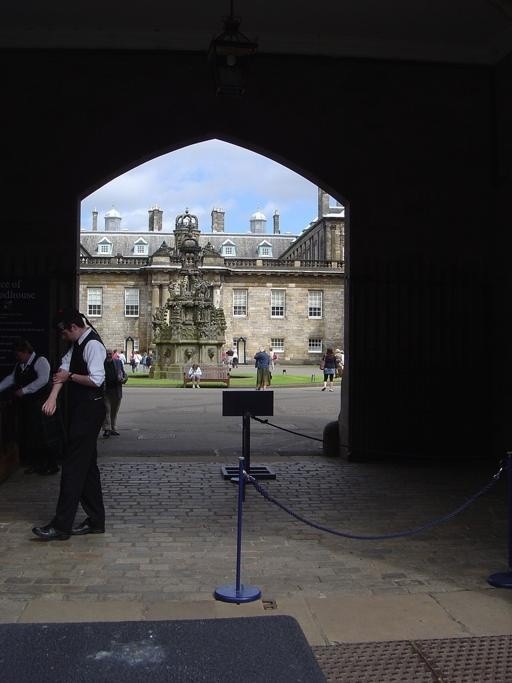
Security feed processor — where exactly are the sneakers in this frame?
[102,430,120,437]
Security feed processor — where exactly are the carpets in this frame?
[0,609,326,682]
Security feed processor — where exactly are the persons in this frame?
[31,306,107,542]
[100,347,153,439]
[0,337,62,476]
[222,347,238,371]
[321,347,344,392]
[188,364,203,389]
[253,346,279,391]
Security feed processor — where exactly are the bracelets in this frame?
[68,372,75,382]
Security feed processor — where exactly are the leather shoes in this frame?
[32,524,70,540]
[71,521,105,535]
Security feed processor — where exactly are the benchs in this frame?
[182,363,232,390]
